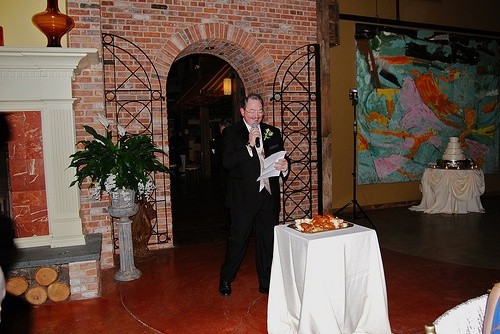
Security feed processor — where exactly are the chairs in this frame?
[425,289,491,334]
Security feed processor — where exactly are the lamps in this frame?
[223,74,235,96]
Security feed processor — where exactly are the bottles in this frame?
[31,0,76,47]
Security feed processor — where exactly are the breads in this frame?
[300,213,343,232]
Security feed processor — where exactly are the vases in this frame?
[109,189,135,209]
[32,0,75,47]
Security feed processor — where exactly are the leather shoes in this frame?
[258,286,269,294]
[219,278,231,296]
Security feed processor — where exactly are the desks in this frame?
[267,220,393,334]
[407,167,486,214]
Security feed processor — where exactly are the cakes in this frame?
[442,137,466,160]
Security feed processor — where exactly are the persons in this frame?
[170,130,187,155]
[168,140,179,190]
[213,93,291,295]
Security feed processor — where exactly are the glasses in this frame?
[243,108,264,116]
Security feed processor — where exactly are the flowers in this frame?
[65,113,176,207]
